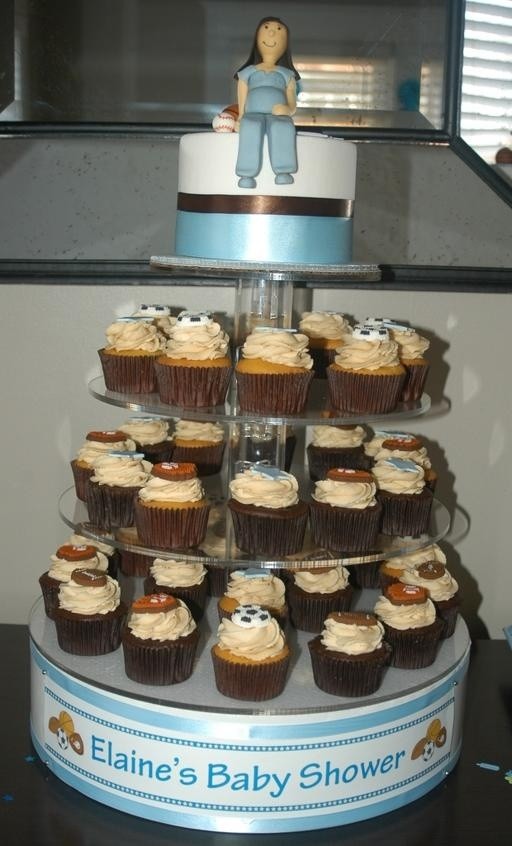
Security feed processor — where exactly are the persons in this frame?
[231,17,302,190]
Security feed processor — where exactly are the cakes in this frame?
[175,16,358,265]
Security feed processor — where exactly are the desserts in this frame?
[39,509,462,700]
[97,302,432,412]
[81,416,439,551]
